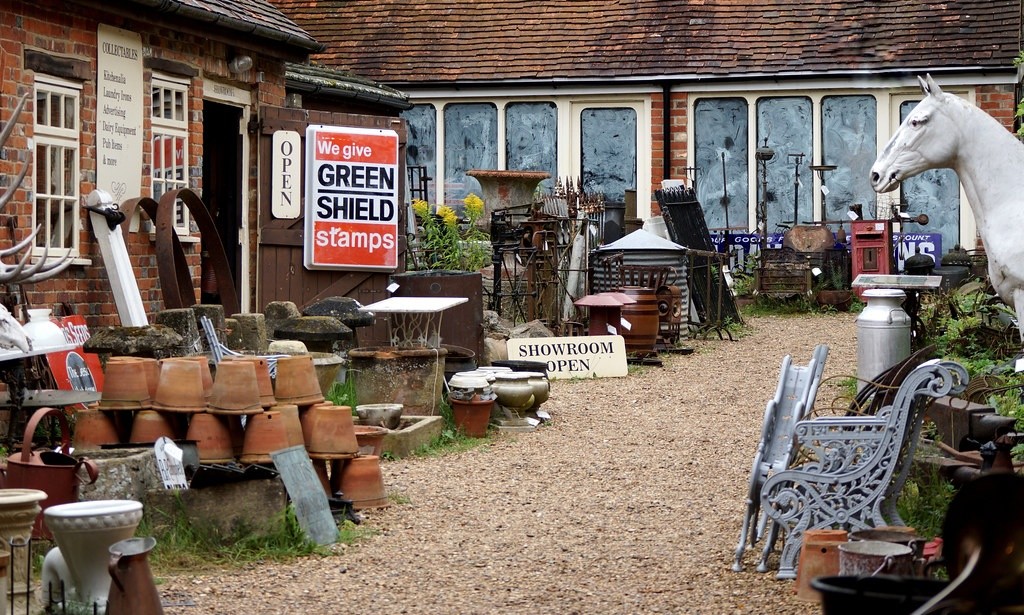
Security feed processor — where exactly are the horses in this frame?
[869,72,1024,358]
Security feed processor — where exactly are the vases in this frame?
[72,355,390,509]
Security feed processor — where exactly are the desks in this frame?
[851,273,942,350]
[358,297,469,347]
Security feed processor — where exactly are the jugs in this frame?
[0,406,99,539]
[103,537,167,615]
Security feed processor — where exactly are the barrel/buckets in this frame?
[932,266,969,294]
[794,524,994,615]
[69,352,391,512]
[448,394,493,439]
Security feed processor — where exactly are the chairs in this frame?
[759,360,969,579]
